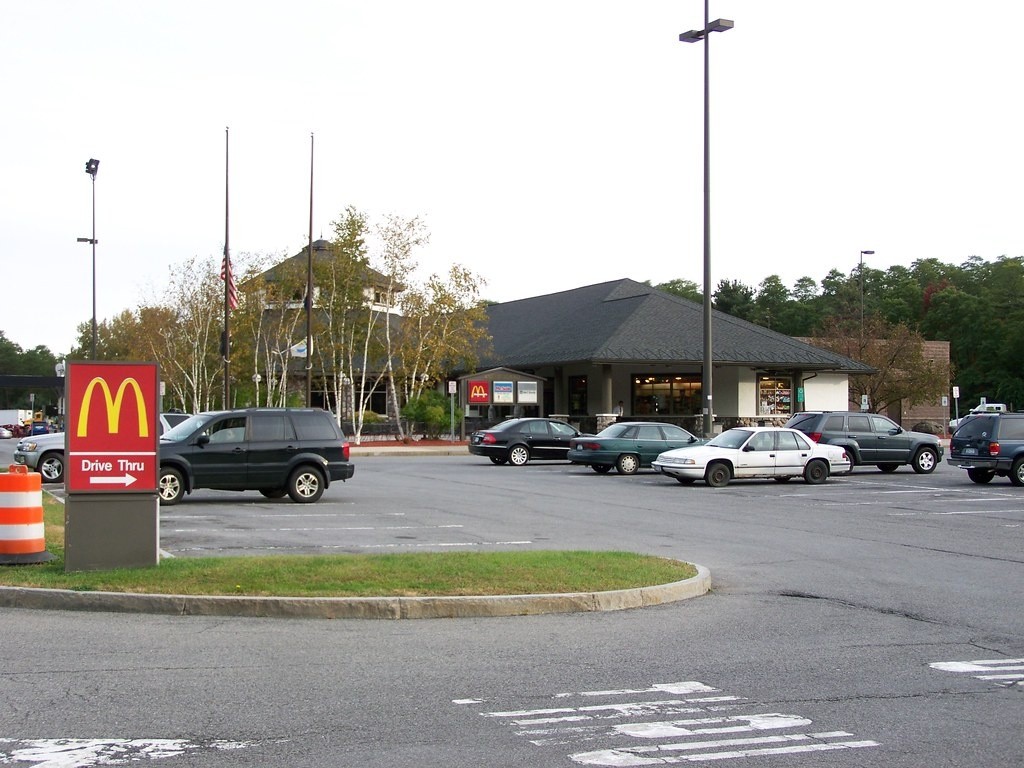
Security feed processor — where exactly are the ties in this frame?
[620,406,622,416]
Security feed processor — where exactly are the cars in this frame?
[14,413,207,485]
[650,427,850,487]
[0,428,12,439]
[566,422,713,474]
[468,419,595,466]
[27,422,49,436]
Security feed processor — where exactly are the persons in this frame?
[613,401,624,417]
[222,426,238,443]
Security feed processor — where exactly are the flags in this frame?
[221,245,240,311]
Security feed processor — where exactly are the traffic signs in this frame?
[65,360,160,494]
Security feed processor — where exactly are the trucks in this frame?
[947,403,1007,434]
[22,410,43,427]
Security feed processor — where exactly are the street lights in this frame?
[77,158,100,362]
[860,250,874,340]
[678,17,735,439]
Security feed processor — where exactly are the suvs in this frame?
[946,410,1024,487]
[159,407,355,506]
[782,411,945,474]
